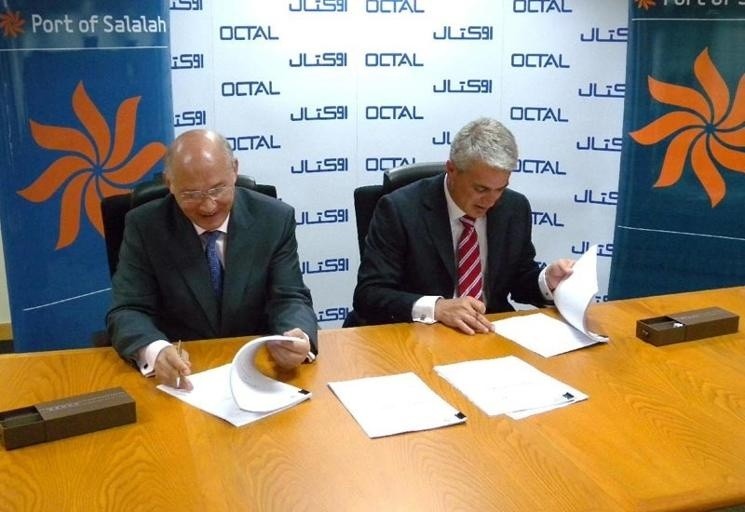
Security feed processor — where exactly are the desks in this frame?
[0,283,743,512]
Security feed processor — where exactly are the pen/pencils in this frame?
[175,339,182,390]
[475,310,481,317]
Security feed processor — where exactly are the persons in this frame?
[352,120,577,335]
[105,130,317,392]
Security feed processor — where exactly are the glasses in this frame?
[181,187,227,204]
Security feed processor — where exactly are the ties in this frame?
[205,231,221,300]
[458,215,483,301]
